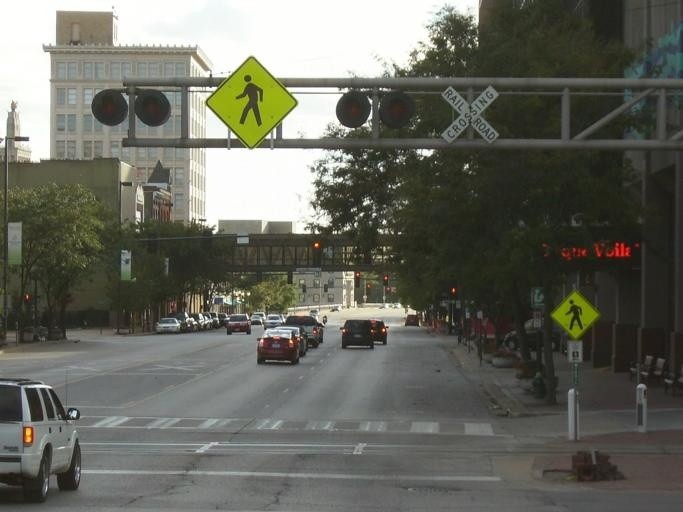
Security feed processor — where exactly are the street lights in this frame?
[2,133,30,350]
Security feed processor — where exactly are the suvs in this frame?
[0,377,82,503]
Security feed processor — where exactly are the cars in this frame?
[155,306,388,364]
[404,314,419,327]
[504,319,559,353]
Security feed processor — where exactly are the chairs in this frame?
[629,353,656,383]
[640,356,668,388]
[664,369,683,399]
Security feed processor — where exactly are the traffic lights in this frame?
[135,92,171,124]
[385,286,390,293]
[367,283,372,288]
[334,93,370,125]
[355,272,360,288]
[448,280,457,299]
[24,293,31,303]
[377,94,415,124]
[92,89,127,126]
[314,242,320,254]
[383,275,388,286]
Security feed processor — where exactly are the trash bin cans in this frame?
[480,334,497,353]
[144,321,153,332]
